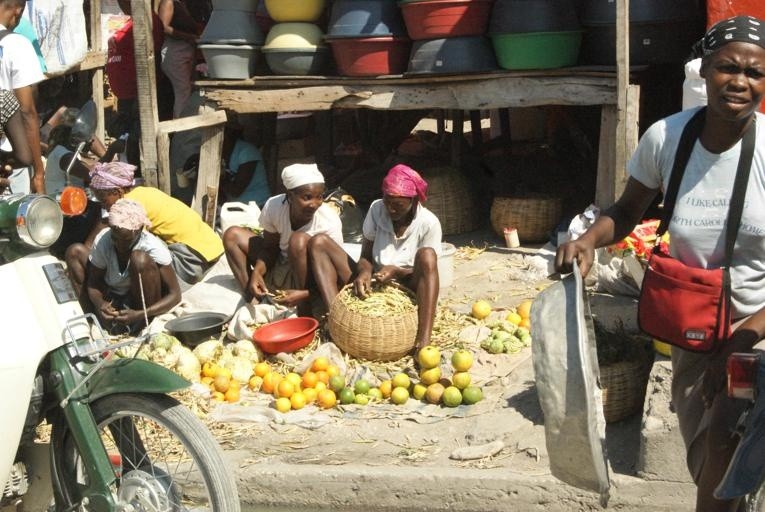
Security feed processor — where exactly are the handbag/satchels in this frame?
[637,103,757,358]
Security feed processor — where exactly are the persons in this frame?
[554,15,765,512]
[106,0,173,186]
[34,75,104,159]
[0,90,34,170]
[45,107,127,260]
[159,2,206,188]
[65,199,181,336]
[223,163,343,318]
[185,120,274,210]
[1,0,42,198]
[89,163,223,292]
[307,166,443,368]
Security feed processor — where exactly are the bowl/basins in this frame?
[194,44,258,80]
[492,35,582,68]
[254,318,319,353]
[326,1,402,37]
[528,260,612,495]
[487,1,580,33]
[164,311,226,347]
[582,0,683,24]
[407,37,489,76]
[329,38,404,76]
[262,50,325,74]
[196,11,262,46]
[403,1,486,36]
[580,24,682,67]
[264,1,322,20]
[260,23,325,49]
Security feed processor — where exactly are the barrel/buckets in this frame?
[0,192,64,252]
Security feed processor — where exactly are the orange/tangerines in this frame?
[201,346,482,413]
[517,302,532,318]
[508,312,522,325]
[520,320,531,330]
[473,296,491,319]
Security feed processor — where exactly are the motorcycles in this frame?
[0,101,241,512]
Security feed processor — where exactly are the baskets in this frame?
[597,335,657,425]
[490,193,566,244]
[328,278,420,363]
[422,162,480,239]
